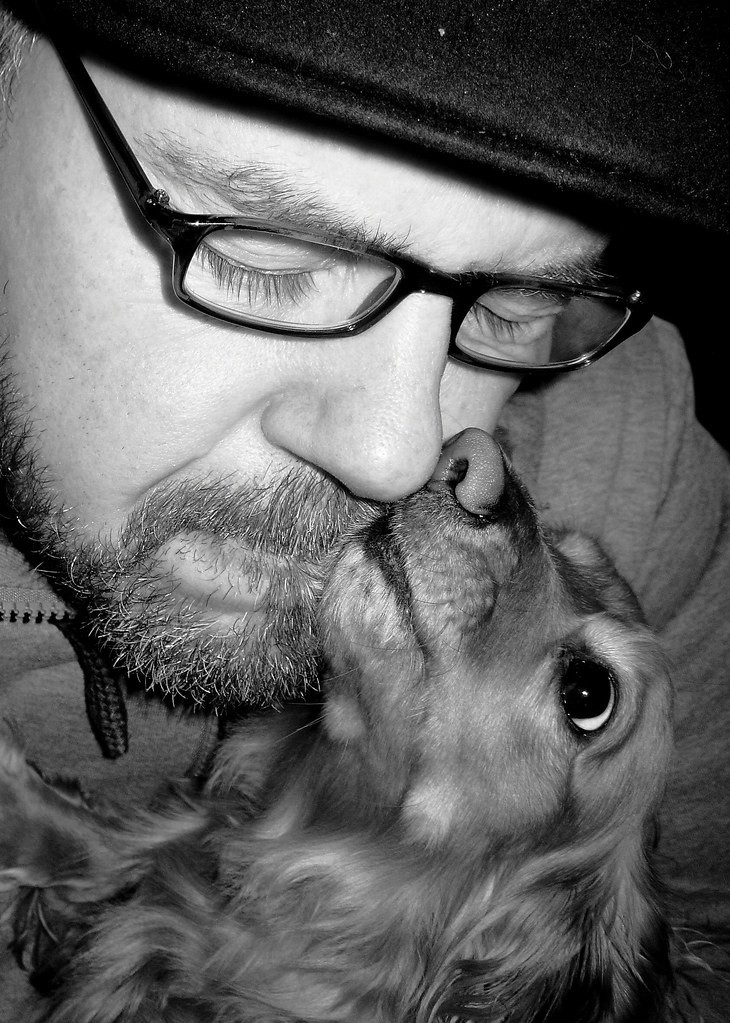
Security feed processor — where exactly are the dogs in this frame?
[1,425,717,1023]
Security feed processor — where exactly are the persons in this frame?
[0,0,730,1023]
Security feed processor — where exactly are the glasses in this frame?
[52,44,650,374]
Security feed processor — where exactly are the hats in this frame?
[0,0,730,229]
